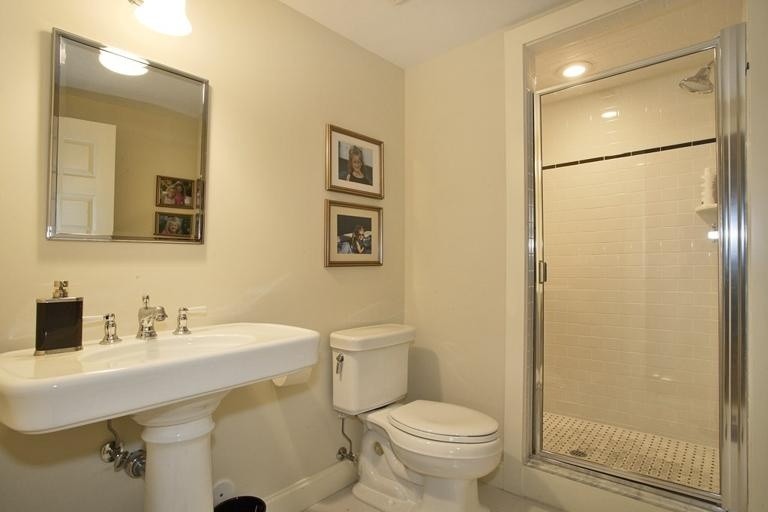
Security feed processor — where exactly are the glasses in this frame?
[356,233,364,236]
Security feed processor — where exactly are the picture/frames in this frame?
[321,198,384,269]
[154,172,195,210]
[324,122,389,201]
[154,212,196,239]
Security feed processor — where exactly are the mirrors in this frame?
[45,28,208,245]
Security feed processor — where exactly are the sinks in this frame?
[0,320,320,437]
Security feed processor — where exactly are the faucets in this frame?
[136,294,168,339]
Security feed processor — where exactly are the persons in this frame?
[163,186,176,205]
[173,182,185,205]
[346,144,371,186]
[161,216,183,235]
[350,225,367,254]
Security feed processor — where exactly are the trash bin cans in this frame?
[213,496,266,512]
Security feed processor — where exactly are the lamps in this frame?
[96,53,147,76]
[132,1,197,39]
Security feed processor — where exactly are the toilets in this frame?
[329,322,503,512]
